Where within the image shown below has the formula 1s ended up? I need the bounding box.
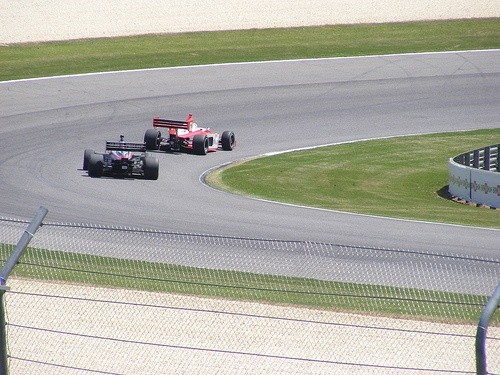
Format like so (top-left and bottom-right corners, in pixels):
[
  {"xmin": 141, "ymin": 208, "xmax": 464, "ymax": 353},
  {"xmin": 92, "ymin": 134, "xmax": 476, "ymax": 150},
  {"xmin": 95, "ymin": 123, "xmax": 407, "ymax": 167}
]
[
  {"xmin": 143, "ymin": 113, "xmax": 236, "ymax": 156},
  {"xmin": 82, "ymin": 134, "xmax": 159, "ymax": 180}
]
